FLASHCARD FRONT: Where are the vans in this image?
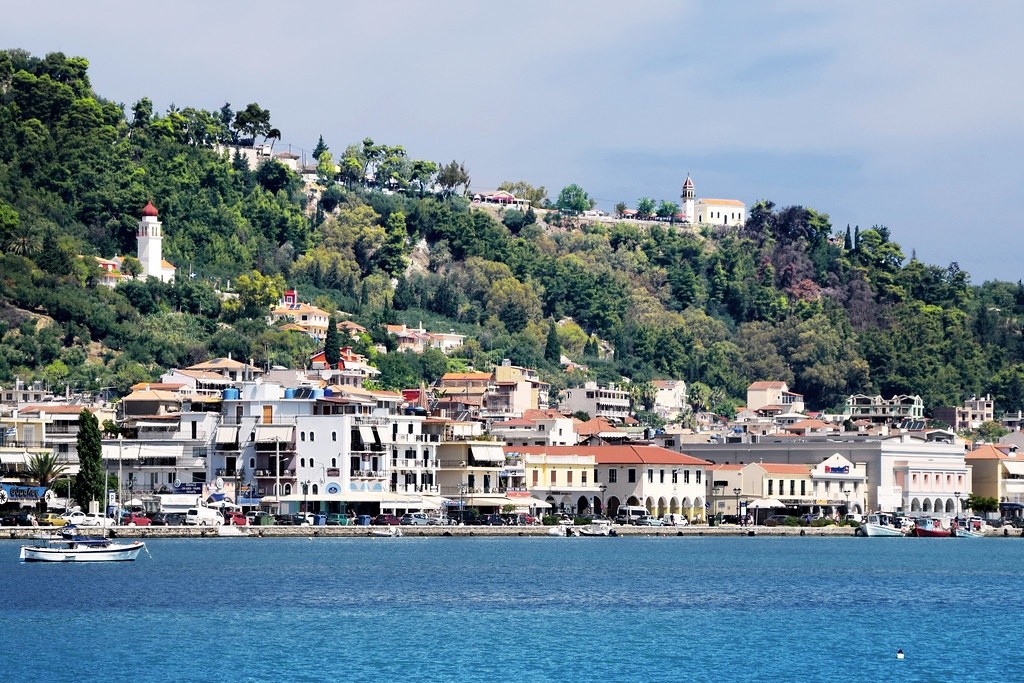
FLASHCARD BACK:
[186,507,225,526]
[664,513,688,527]
[616,505,651,526]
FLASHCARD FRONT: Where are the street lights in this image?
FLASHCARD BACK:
[844,490,850,512]
[733,488,741,525]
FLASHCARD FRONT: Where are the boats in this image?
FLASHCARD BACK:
[859,509,1024,539]
[18,469,146,562]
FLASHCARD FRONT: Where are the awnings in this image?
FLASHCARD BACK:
[999,503,1024,511]
[424,497,552,508]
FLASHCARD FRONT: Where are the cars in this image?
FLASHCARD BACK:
[429,513,457,526]
[374,513,401,526]
[763,514,789,526]
[637,515,665,526]
[37,513,69,526]
[122,512,152,526]
[448,509,616,526]
[800,512,863,526]
[60,511,86,526]
[83,512,117,527]
[245,511,274,525]
[400,513,431,526]
[151,513,184,526]
[275,512,352,526]
[224,512,253,525]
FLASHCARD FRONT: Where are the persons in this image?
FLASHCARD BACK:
[805,514,811,527]
[345,508,356,525]
[25,512,39,526]
[696,512,701,523]
[739,513,755,527]
[835,514,841,526]
[670,512,675,526]
